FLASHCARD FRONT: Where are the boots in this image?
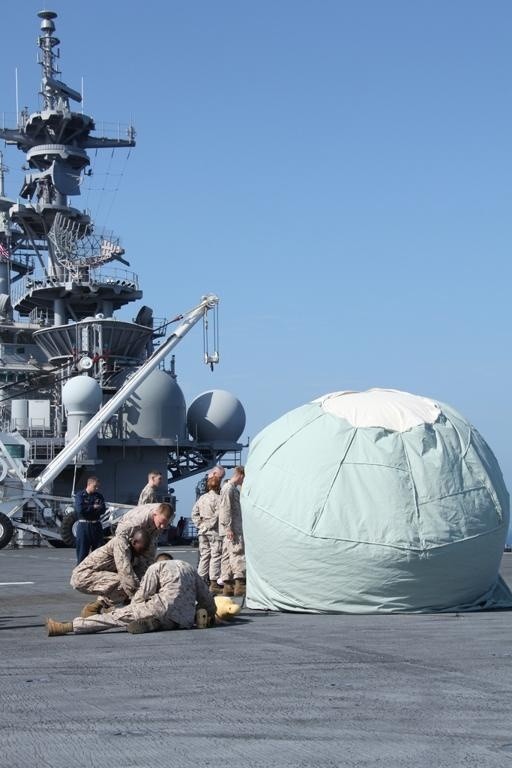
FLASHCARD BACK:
[127,617,161,634]
[46,616,73,636]
[80,601,101,618]
[209,580,247,596]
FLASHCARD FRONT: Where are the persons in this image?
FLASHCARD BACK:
[71,475,106,567]
[219,464,247,599]
[192,466,227,571]
[68,527,152,620]
[43,552,218,638]
[189,476,225,595]
[111,501,175,564]
[137,470,164,505]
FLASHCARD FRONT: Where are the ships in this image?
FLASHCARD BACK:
[0,8,512,768]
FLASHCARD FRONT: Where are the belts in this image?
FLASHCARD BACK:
[79,519,101,524]
